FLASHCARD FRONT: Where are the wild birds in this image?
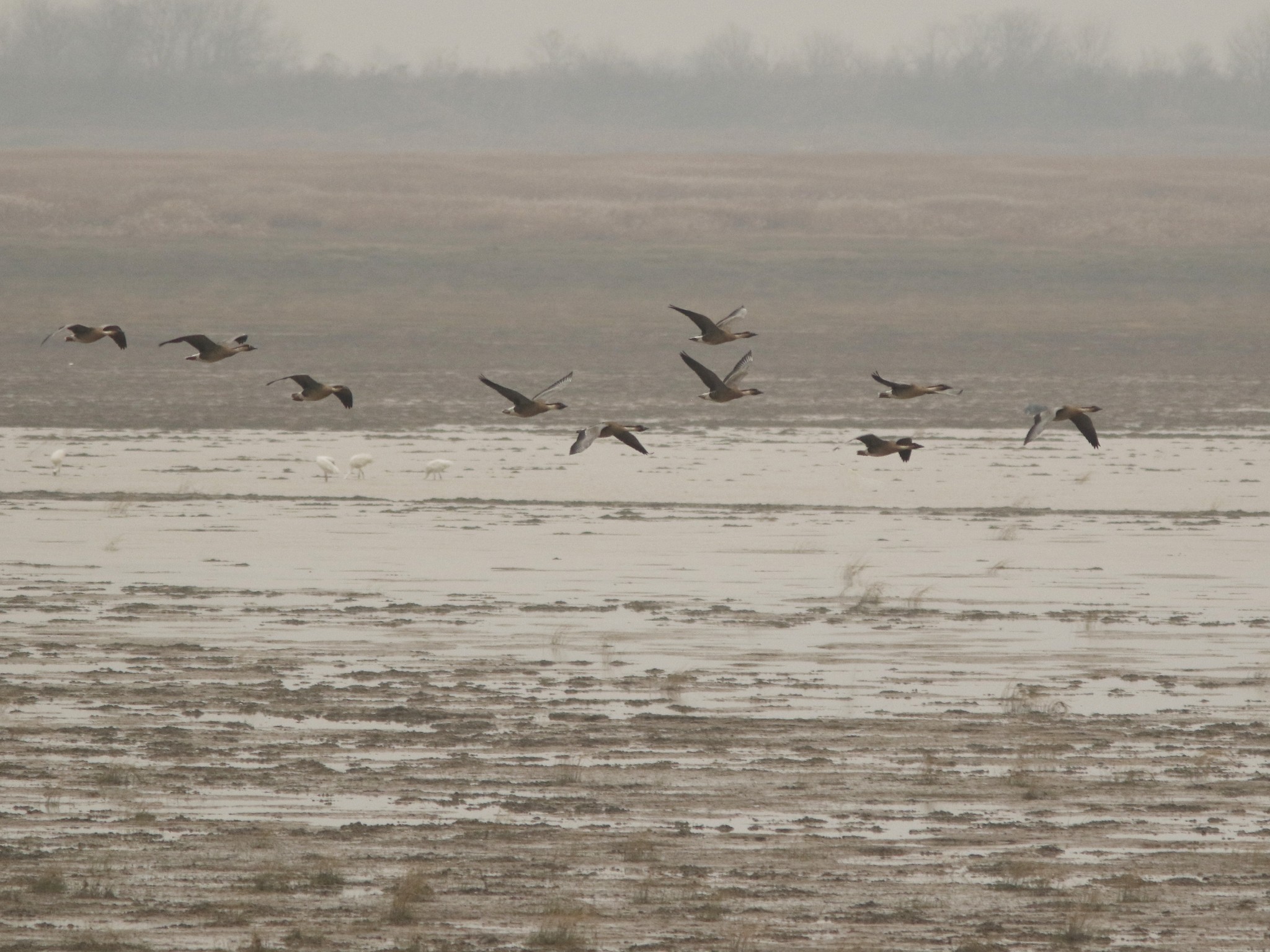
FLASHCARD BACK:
[478,371,573,418]
[835,433,923,461]
[569,421,653,455]
[870,371,965,398]
[680,350,763,402]
[159,334,257,362]
[1021,402,1104,448]
[316,453,375,481]
[51,449,64,476]
[266,374,353,409]
[41,323,128,350]
[668,304,758,345]
[423,459,454,479]
